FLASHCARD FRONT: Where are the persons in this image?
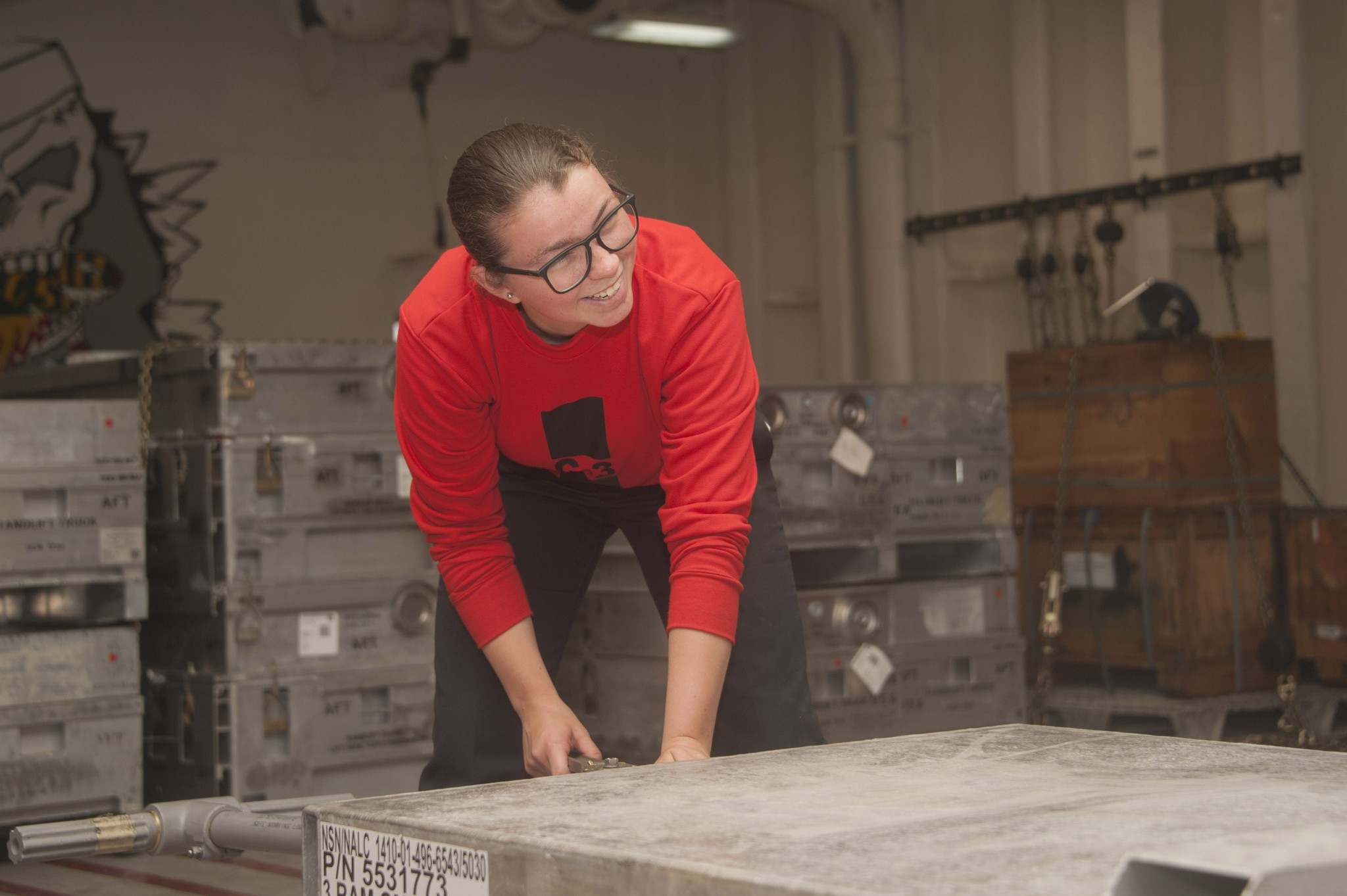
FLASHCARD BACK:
[398,125,815,791]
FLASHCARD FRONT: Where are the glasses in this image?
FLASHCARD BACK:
[500,187,638,294]
